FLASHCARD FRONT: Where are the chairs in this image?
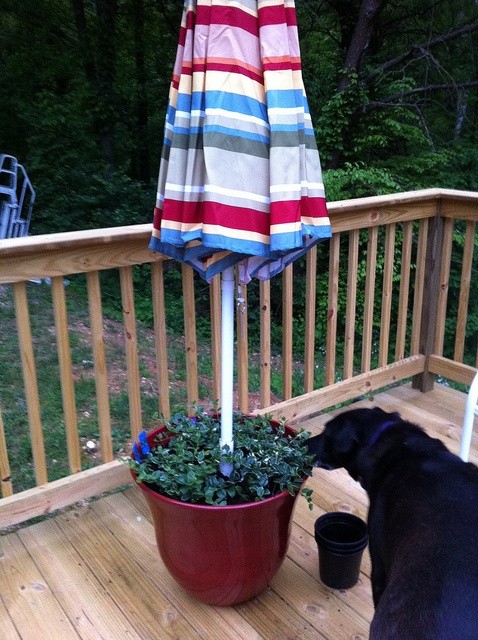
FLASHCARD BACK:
[460,371,478,464]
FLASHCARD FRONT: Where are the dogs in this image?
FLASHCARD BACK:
[300,408,478,640]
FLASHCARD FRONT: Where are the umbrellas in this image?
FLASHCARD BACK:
[147,1,333,480]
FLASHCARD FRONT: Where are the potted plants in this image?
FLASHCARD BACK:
[120,384,324,608]
